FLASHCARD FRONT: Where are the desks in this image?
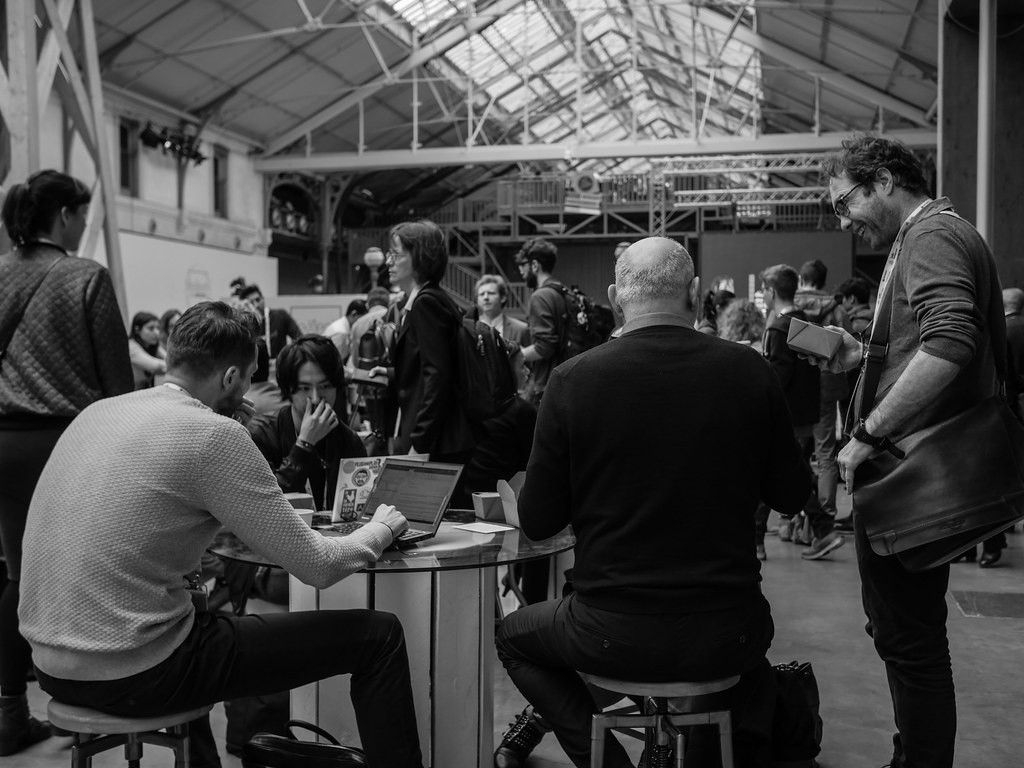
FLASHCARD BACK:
[204,503,576,768]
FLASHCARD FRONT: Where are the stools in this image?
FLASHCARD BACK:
[579,670,742,768]
[46,697,213,768]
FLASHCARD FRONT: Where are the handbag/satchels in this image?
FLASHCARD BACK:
[853,395,1023,569]
[691,662,822,768]
[244,720,368,768]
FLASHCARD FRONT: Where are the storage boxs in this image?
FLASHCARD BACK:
[471,469,526,529]
[780,317,843,362]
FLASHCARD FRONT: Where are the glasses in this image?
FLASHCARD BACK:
[385,251,411,263]
[834,178,867,219]
[518,258,530,269]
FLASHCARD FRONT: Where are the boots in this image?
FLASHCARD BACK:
[1,694,52,757]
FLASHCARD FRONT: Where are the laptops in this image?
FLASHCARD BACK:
[306,452,466,552]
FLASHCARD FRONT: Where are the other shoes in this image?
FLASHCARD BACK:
[225,739,245,758]
[755,545,768,562]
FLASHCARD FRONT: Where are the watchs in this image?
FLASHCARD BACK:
[852,418,905,460]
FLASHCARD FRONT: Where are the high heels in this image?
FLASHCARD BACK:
[953,546,978,563]
[502,573,520,596]
[980,532,1008,568]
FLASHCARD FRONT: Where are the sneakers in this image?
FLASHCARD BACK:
[801,531,846,560]
[494,704,551,768]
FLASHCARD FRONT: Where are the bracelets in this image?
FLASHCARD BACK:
[296,439,314,450]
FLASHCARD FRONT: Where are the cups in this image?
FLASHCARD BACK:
[736,340,751,347]
[295,508,314,529]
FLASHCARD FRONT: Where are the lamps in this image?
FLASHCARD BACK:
[139,121,207,166]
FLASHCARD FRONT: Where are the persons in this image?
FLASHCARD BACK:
[322,286,397,370]
[495,238,818,768]
[951,287,1024,567]
[465,273,528,343]
[248,333,369,511]
[821,135,1024,768]
[0,169,135,756]
[514,238,576,405]
[383,219,484,508]
[15,301,424,768]
[699,259,874,560]
[127,310,182,391]
[240,285,302,383]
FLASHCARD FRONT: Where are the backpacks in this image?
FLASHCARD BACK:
[546,284,613,351]
[418,289,517,421]
